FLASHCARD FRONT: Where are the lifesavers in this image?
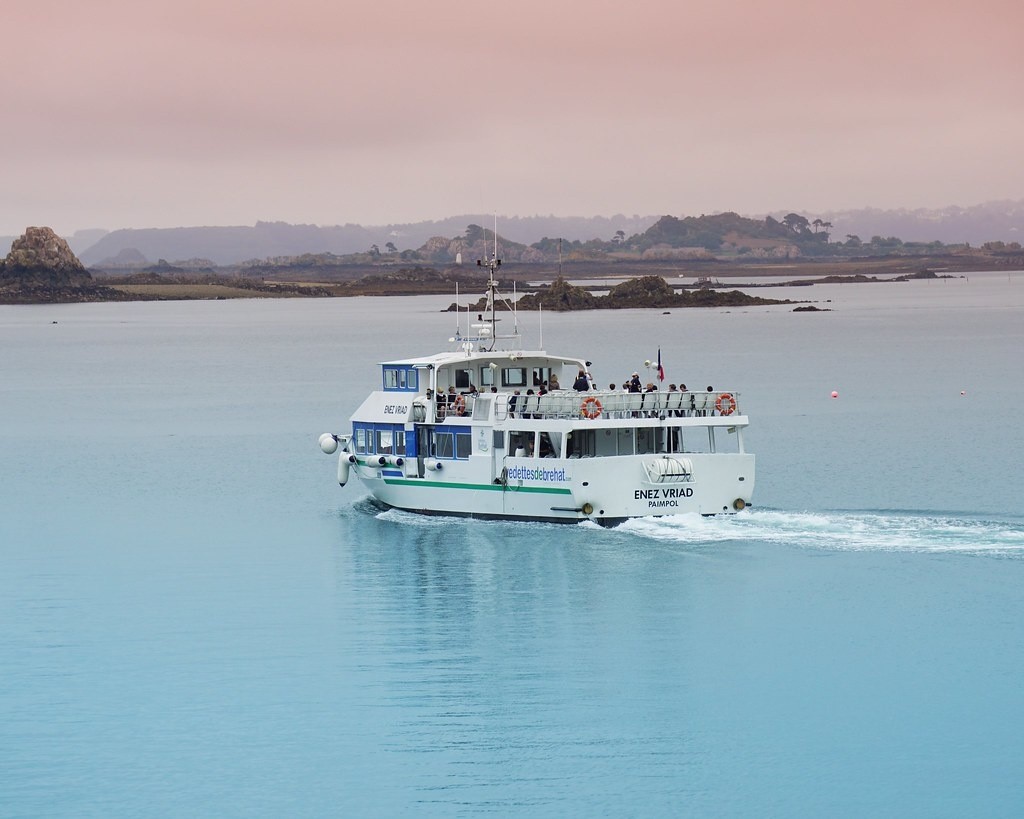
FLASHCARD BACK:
[454,396,466,415]
[581,397,602,418]
[715,393,736,415]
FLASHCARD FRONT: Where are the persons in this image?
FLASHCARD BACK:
[437,369,718,458]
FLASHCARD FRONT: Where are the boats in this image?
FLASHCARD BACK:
[317,210,757,531]
